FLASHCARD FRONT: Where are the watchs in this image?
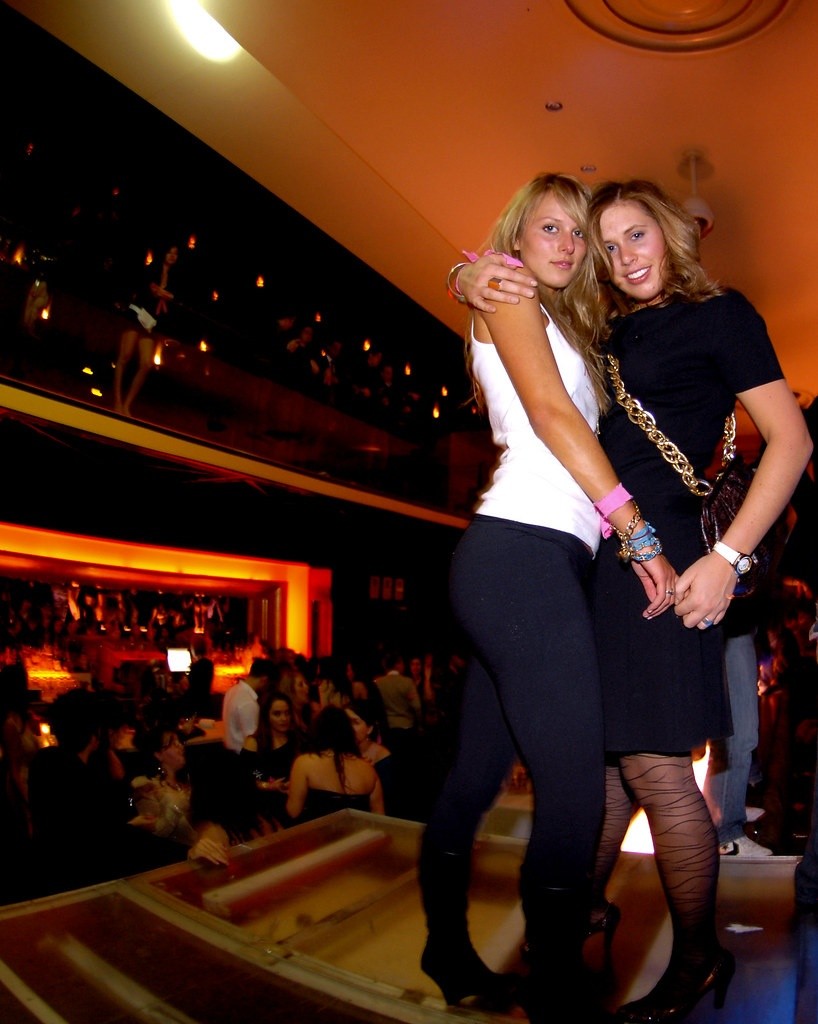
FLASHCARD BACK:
[712,541,752,576]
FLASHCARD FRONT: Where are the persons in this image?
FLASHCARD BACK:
[109,245,418,465]
[455,178,813,1024]
[0,640,421,907]
[418,173,690,1024]
[703,577,817,857]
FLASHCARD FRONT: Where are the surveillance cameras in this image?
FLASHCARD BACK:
[678,197,715,234]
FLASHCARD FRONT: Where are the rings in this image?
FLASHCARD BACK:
[488,278,501,291]
[702,617,712,626]
[666,591,674,595]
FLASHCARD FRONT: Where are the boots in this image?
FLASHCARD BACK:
[418,842,523,1005]
[517,891,624,1024]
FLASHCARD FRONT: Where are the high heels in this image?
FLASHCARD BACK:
[520,903,621,954]
[615,952,735,1024]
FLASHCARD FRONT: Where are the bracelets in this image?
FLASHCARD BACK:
[613,500,662,563]
[594,482,633,539]
[448,263,467,303]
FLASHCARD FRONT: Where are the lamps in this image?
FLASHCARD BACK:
[679,148,715,231]
[564,0,788,54]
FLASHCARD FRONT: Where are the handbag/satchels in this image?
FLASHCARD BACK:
[701,454,790,599]
